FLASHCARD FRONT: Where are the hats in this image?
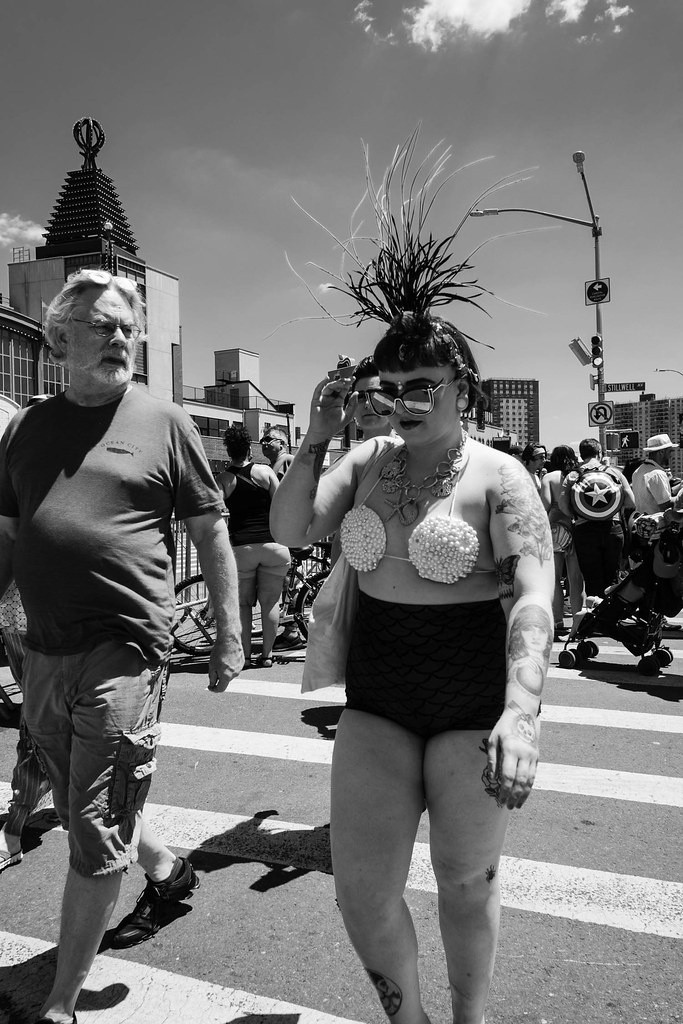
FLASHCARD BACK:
[643,434,679,452]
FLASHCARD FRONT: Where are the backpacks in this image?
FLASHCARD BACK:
[568,463,626,521]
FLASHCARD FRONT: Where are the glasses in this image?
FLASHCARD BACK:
[66,316,141,341]
[88,270,138,291]
[260,437,282,444]
[365,374,469,417]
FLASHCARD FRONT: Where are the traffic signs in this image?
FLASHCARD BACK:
[607,382,646,392]
[585,278,610,305]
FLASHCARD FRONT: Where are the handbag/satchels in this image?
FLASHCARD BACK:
[302,436,396,693]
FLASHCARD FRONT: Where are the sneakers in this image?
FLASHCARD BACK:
[112,856,200,950]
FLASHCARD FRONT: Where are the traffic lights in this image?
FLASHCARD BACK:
[591,334,604,368]
[619,432,640,450]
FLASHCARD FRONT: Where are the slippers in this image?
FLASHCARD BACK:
[0,848,24,871]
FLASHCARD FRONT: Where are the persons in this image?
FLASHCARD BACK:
[270,316,554,1024]
[214,424,292,669]
[511,432,683,642]
[0,268,247,1024]
[260,428,303,650]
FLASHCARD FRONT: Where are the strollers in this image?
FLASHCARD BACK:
[558,520,683,676]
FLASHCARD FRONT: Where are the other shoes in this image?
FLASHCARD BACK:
[553,620,563,631]
[242,657,252,668]
[273,633,303,651]
[257,654,274,667]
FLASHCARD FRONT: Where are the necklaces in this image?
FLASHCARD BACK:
[381,428,469,527]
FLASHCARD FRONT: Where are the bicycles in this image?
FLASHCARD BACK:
[171,541,332,657]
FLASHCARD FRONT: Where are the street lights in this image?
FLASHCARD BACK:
[468,150,607,458]
